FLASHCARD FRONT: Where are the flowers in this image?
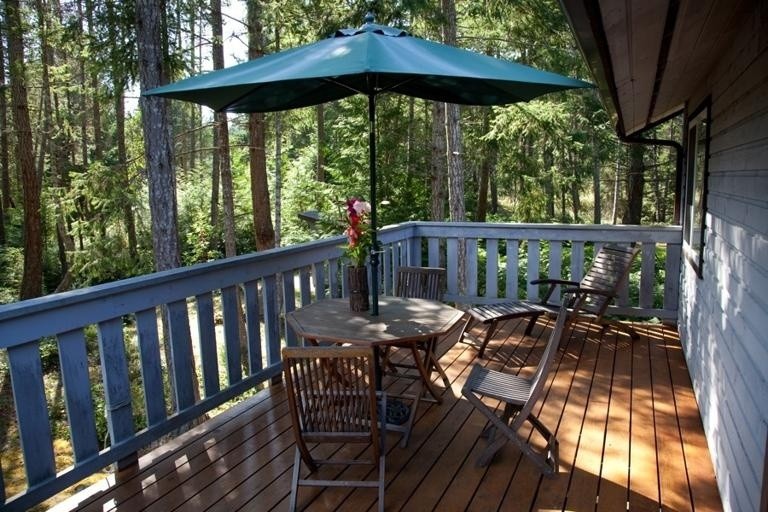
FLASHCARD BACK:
[343,195,373,268]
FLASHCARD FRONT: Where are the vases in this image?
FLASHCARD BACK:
[346,265,370,312]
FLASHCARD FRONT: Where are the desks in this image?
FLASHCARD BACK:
[284,294,465,450]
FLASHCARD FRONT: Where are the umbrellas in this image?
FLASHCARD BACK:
[135,13,597,400]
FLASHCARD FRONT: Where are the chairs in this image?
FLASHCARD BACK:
[461,305,567,481]
[376,266,448,401]
[458,245,642,358]
[282,344,387,512]
